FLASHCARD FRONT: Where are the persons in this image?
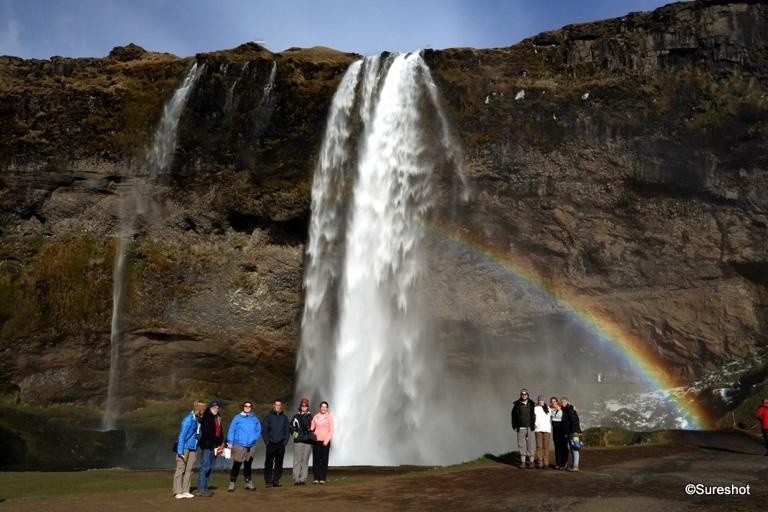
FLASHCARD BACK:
[560,399,581,472]
[754,397,767,457]
[289,399,313,485]
[510,389,534,469]
[225,400,262,492]
[532,396,551,469]
[260,399,290,488]
[197,401,219,498]
[312,402,333,484]
[548,397,576,470]
[172,401,207,499]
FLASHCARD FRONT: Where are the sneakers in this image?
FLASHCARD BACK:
[174,480,326,499]
[518,462,579,472]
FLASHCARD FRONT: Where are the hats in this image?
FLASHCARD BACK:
[210,399,309,407]
[519,388,569,401]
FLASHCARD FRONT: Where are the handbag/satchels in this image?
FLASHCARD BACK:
[172,441,178,452]
